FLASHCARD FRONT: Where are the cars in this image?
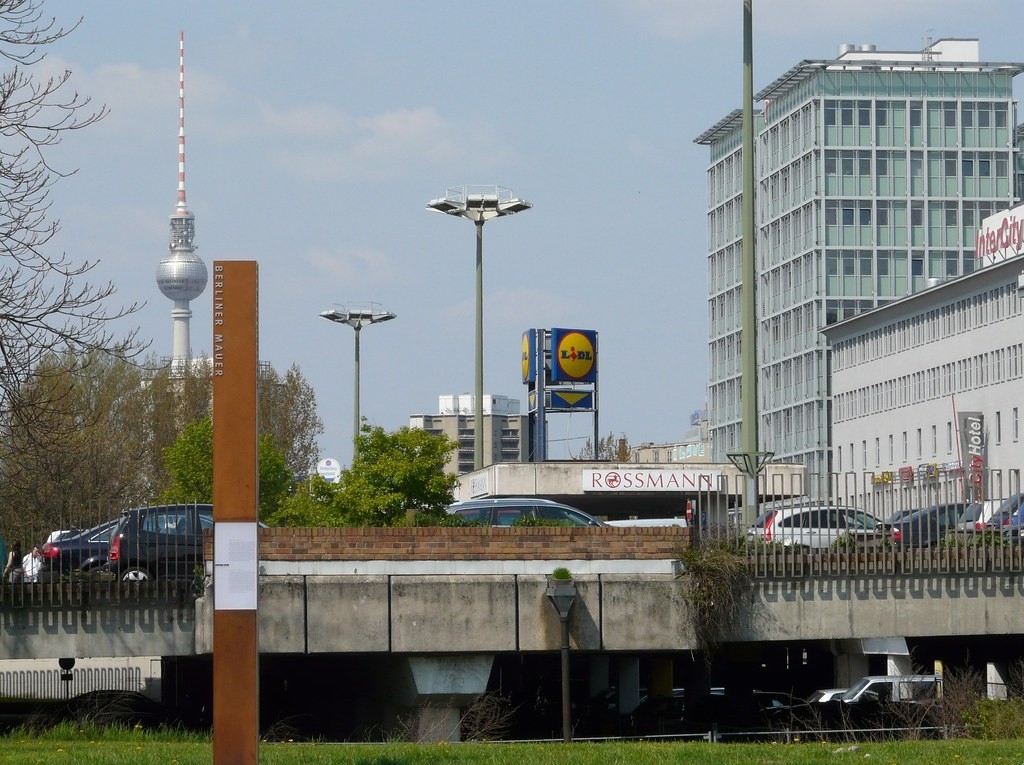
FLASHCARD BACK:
[438,499,621,528]
[747,495,1024,544]
[594,675,945,732]
[40,502,271,586]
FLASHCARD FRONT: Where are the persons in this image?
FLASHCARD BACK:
[23,546,42,582]
[3,538,24,582]
